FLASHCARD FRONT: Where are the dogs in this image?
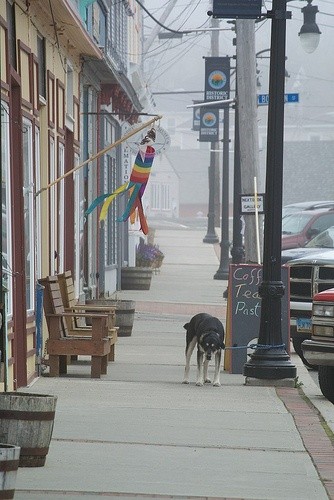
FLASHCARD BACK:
[183,312,225,386]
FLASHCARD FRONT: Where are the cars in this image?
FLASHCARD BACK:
[334,226,334,266]
[284,250,334,372]
[282,201,334,217]
[302,287,334,401]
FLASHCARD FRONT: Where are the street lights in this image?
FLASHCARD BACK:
[224,49,288,299]
[244,1,324,379]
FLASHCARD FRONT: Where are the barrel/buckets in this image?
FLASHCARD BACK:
[121,267,153,290]
[0,392,58,467]
[0,442,21,500]
[86,300,136,337]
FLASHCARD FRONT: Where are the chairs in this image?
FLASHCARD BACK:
[39,269,119,380]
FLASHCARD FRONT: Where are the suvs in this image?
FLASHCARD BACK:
[282,211,334,248]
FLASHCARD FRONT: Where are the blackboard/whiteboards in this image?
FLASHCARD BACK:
[225,263,290,375]
